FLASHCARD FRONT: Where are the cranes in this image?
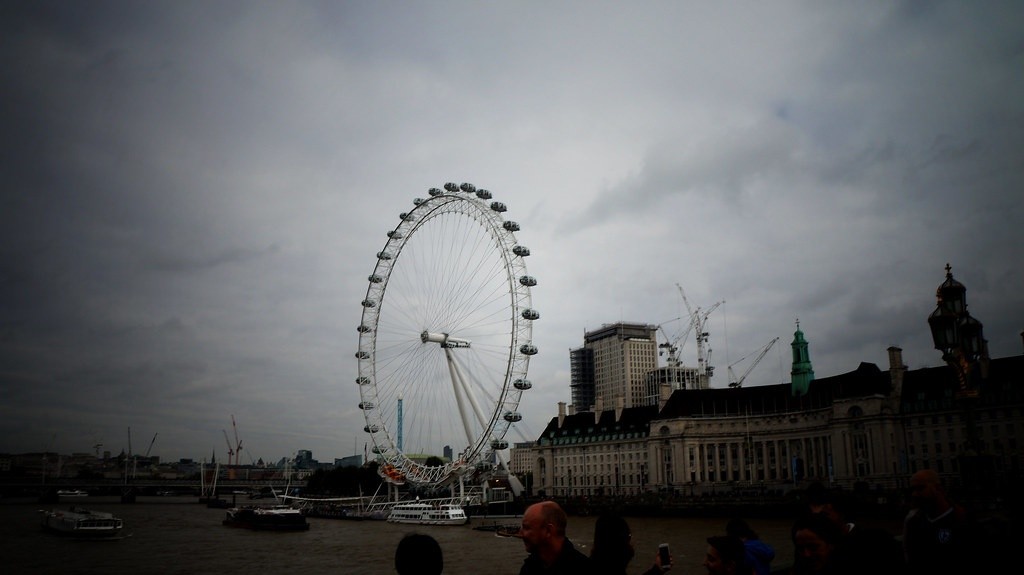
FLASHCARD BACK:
[656,283,779,394]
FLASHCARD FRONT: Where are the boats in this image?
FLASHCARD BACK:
[469,501,529,530]
[387,496,468,525]
[222,504,310,532]
[35,507,134,542]
[269,483,397,521]
[57,489,88,503]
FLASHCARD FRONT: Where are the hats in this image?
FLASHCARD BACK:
[911,469,940,497]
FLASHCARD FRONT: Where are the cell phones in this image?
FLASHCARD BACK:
[659,543,672,569]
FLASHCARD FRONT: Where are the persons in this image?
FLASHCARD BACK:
[703,518,776,575]
[588,515,675,575]
[395,534,443,575]
[790,481,908,575]
[901,470,974,575]
[517,500,602,575]
